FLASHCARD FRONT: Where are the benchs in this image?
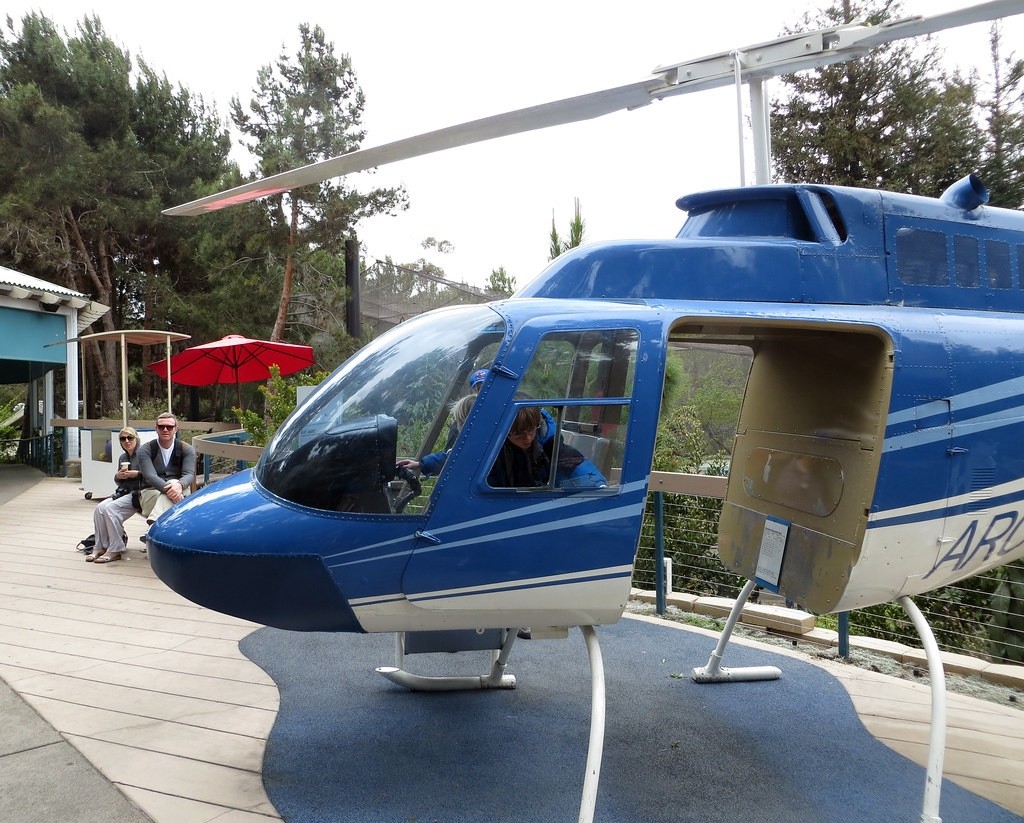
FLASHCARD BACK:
[192,473,232,492]
[559,427,616,481]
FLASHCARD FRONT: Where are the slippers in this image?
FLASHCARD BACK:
[94,555,121,563]
[86,552,102,562]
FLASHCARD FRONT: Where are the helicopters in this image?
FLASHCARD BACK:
[140,0,1022,823]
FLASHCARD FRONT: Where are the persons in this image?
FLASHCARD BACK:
[84,426,144,564]
[390,367,613,489]
[135,412,197,551]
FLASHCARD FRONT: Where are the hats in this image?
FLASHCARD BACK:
[469,369,490,389]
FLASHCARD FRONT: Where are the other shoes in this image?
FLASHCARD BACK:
[140,535,146,543]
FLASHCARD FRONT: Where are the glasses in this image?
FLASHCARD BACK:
[120,436,134,441]
[507,424,542,439]
[157,425,175,431]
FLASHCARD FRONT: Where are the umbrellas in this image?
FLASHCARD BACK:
[147,331,316,428]
